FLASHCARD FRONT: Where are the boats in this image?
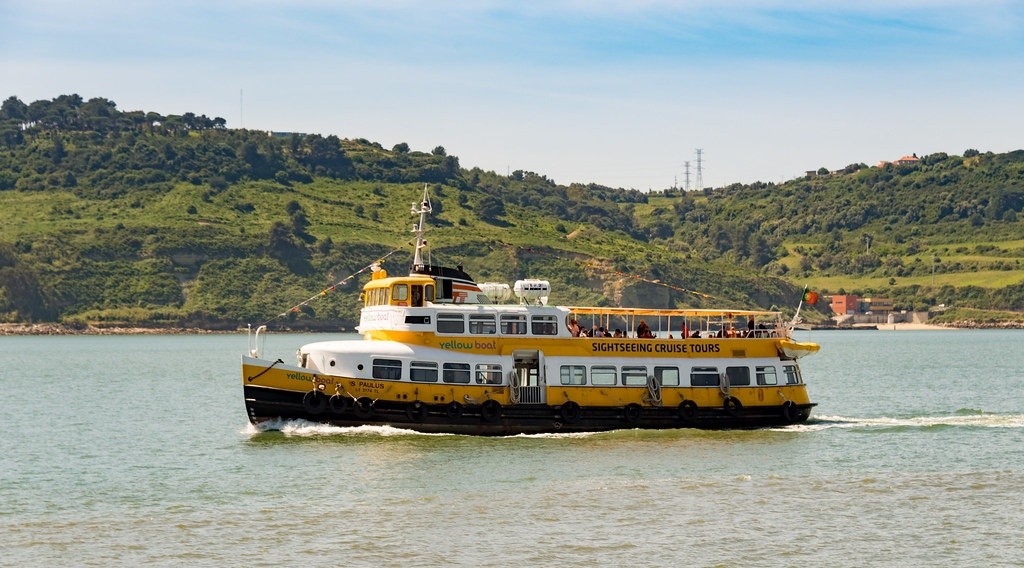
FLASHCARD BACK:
[242,184,820,437]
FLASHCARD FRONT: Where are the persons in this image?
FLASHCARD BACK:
[717,325,735,338]
[742,317,766,338]
[691,331,701,338]
[636,320,657,339]
[681,322,689,339]
[570,319,629,338]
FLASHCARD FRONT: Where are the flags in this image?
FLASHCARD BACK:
[802,289,819,304]
